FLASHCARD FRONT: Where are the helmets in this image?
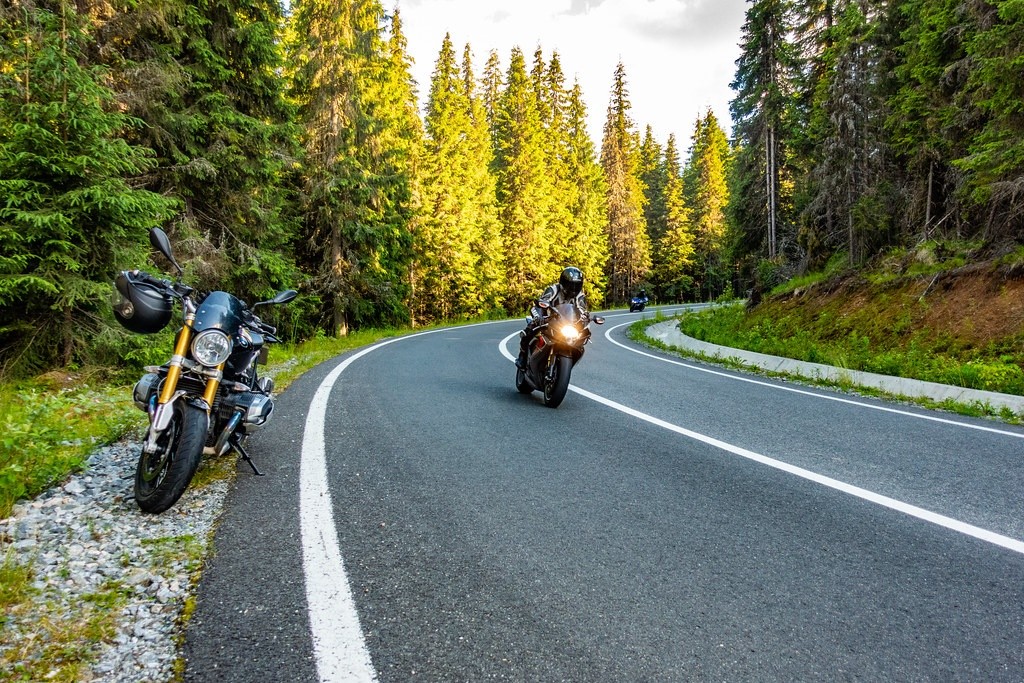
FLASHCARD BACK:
[113,271,173,334]
[641,289,645,293]
[560,267,584,298]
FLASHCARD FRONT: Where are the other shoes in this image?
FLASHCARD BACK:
[516,356,528,371]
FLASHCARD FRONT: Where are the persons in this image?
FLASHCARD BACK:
[637,289,646,309]
[517,267,591,371]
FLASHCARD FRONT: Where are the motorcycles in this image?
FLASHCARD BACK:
[514,297,605,408]
[134,227,299,514]
[629,296,649,313]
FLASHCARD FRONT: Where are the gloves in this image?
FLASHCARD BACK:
[534,315,544,326]
